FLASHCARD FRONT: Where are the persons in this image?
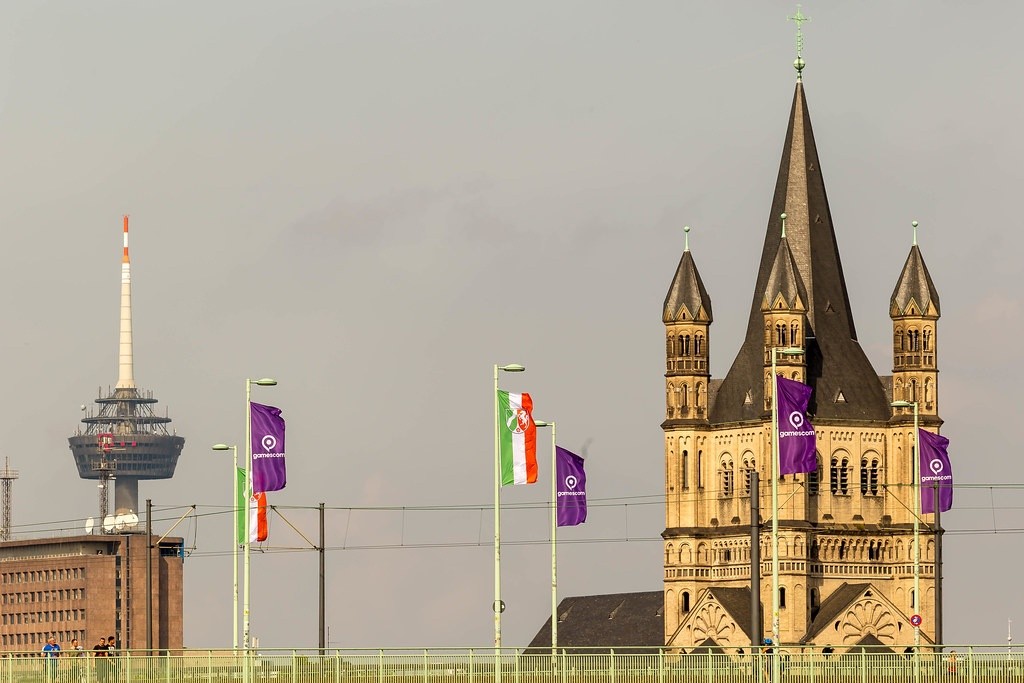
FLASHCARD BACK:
[69,636,119,683]
[947,651,958,676]
[41,637,61,683]
[761,639,781,683]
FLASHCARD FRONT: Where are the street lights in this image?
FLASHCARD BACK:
[535,419,557,668]
[493,363,526,682]
[242,378,277,683]
[212,444,239,657]
[771,345,805,683]
[890,401,920,682]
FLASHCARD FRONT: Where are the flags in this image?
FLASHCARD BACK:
[236,467,267,545]
[249,401,287,496]
[919,428,953,515]
[497,389,538,488]
[776,374,817,476]
[556,445,587,527]
